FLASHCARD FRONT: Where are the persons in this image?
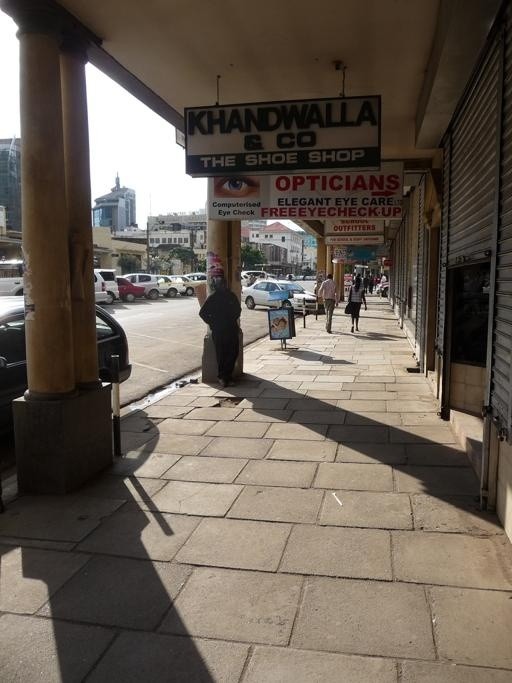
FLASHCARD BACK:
[318,274,341,332]
[198,276,243,388]
[271,317,279,328]
[344,270,386,294]
[271,316,287,332]
[213,174,260,198]
[348,275,367,332]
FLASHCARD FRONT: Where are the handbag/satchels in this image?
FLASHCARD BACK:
[345,302,351,313]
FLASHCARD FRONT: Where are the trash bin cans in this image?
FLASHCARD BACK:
[267,290,296,350]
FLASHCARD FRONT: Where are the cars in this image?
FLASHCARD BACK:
[0,259,206,304]
[241,279,318,313]
[241,271,316,286]
[0,301,132,419]
